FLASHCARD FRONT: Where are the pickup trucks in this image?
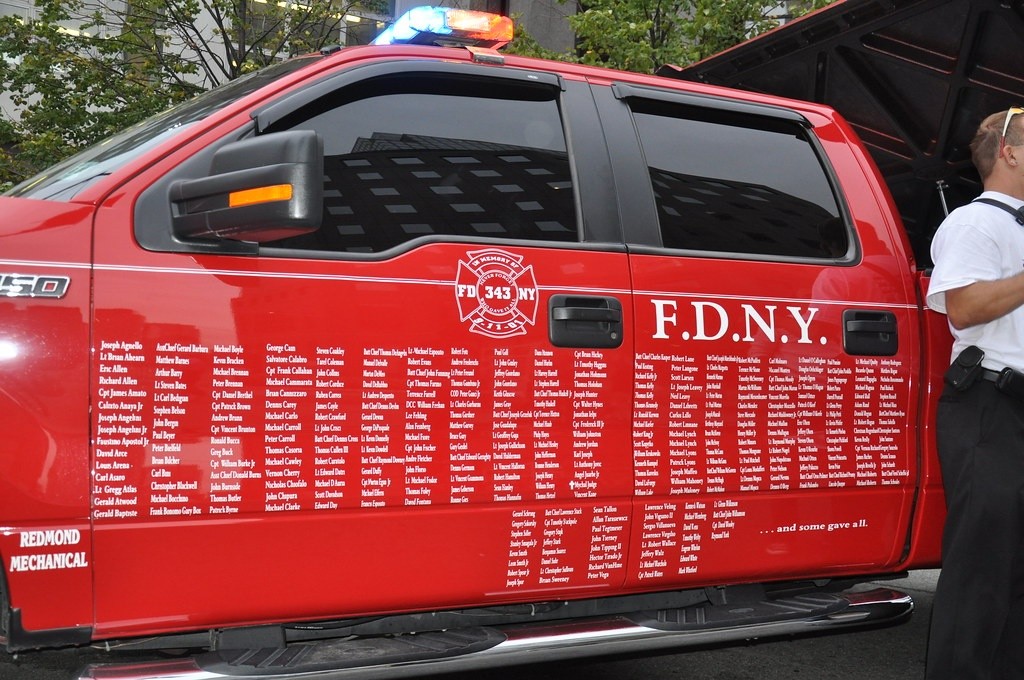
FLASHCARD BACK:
[0,4,953,680]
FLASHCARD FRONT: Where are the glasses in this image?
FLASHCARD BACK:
[998,106,1024,158]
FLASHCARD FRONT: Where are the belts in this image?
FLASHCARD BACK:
[976,369,1000,381]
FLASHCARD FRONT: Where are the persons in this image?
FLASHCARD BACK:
[920,103,1023,680]
